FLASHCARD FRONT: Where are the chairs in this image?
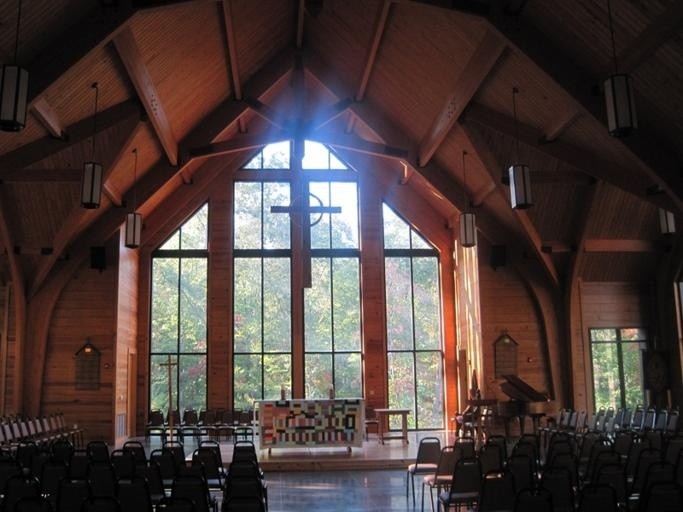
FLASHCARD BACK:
[366,404,381,442]
[0,409,269,512]
[404,401,683,512]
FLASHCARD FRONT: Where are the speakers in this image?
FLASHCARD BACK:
[492,244,505,266]
[90,247,105,270]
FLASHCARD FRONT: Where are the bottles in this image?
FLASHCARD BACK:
[329,384,334,399]
[281,385,286,400]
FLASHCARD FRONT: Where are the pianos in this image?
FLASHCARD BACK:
[498,375,551,445]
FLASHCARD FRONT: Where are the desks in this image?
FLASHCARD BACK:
[253,398,366,447]
[374,407,412,446]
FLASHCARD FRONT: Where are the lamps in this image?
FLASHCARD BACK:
[458,148,480,248]
[602,0,640,137]
[505,86,536,211]
[78,82,105,210]
[655,204,677,238]
[0,0,30,134]
[123,147,143,249]
